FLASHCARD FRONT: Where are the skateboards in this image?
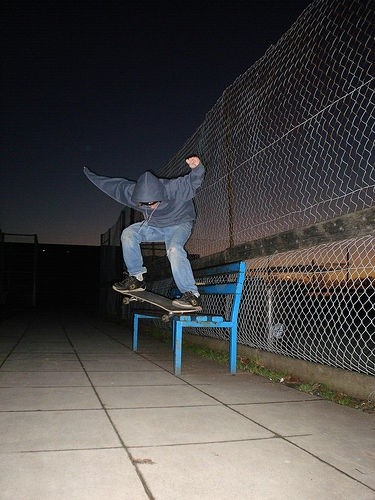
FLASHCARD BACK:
[113,283,202,322]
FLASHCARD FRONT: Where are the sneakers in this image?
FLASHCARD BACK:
[112,272,147,294]
[172,291,203,311]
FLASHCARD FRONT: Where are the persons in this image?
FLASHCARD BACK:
[82,156,207,310]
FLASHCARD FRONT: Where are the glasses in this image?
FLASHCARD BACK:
[138,202,160,207]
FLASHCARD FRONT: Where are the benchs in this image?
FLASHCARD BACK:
[132,261,247,376]
